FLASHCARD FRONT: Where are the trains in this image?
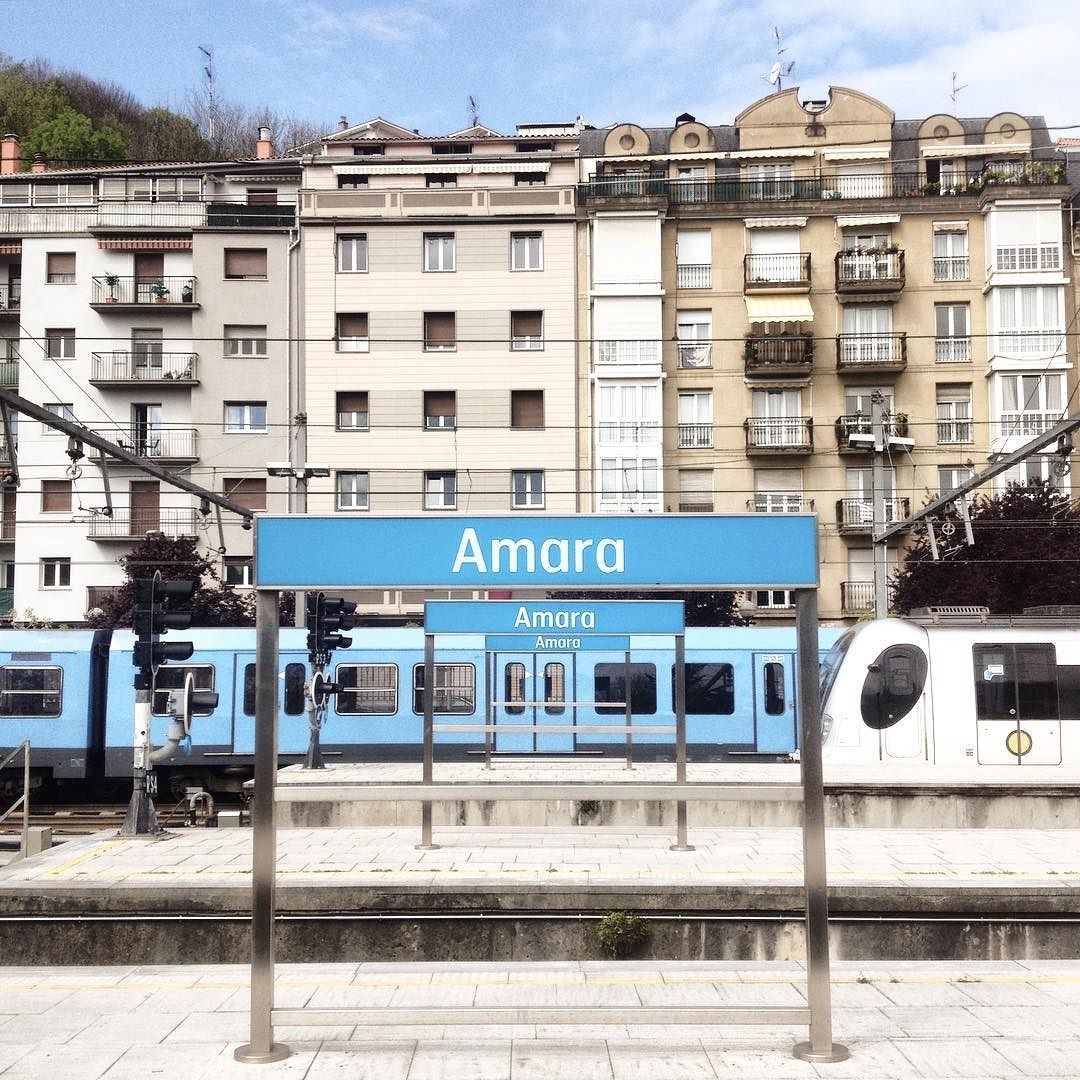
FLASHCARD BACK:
[0,626,844,806]
[798,617,1080,793]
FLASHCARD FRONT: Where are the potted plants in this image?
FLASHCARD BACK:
[105,273,119,304]
[182,280,193,302]
[150,281,170,303]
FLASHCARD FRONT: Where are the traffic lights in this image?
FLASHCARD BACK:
[342,602,359,649]
[150,570,195,677]
[311,671,345,708]
[315,592,346,650]
[183,673,219,735]
